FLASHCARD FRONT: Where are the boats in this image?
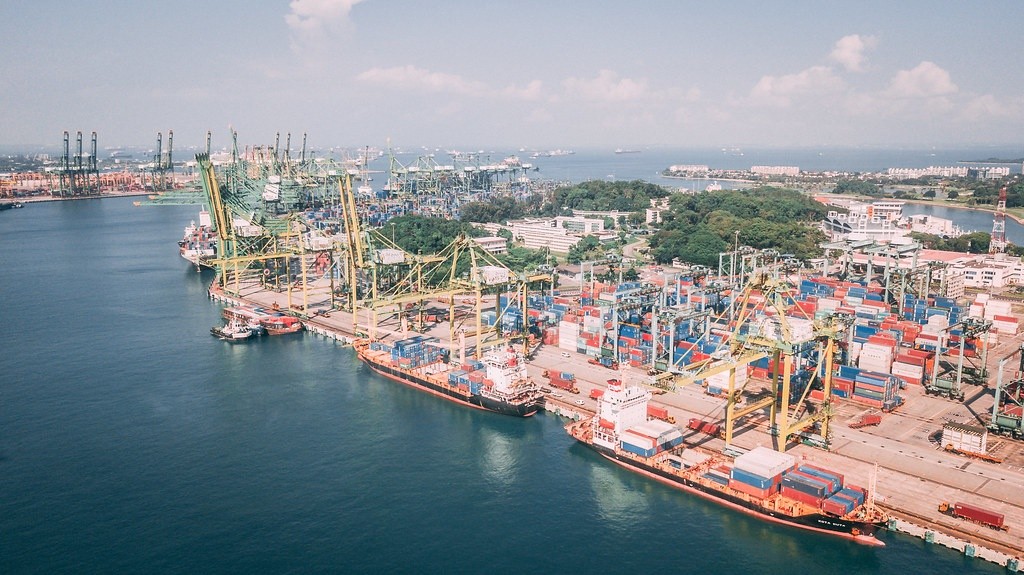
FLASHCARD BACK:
[563,374,890,549]
[351,335,547,417]
[209,307,301,344]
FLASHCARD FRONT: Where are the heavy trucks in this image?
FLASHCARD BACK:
[939,500,1010,533]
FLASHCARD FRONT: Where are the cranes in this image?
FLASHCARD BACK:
[602,242,1024,453]
[54,128,520,306]
